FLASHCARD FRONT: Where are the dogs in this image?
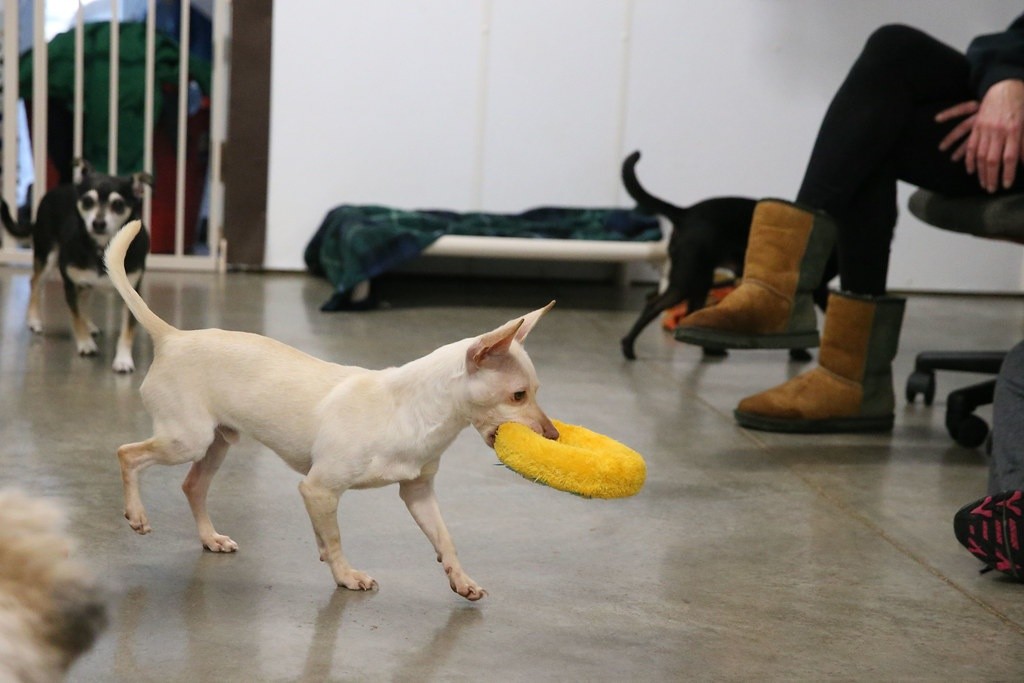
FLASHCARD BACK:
[622,148,850,365]
[103,217,560,604]
[0,156,156,373]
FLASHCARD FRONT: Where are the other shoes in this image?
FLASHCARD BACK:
[953,490,1024,583]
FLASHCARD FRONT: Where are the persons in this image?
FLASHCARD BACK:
[954,341,1024,580]
[675,11,1024,432]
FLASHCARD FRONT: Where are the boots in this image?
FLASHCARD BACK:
[674,197,838,349]
[735,289,908,435]
